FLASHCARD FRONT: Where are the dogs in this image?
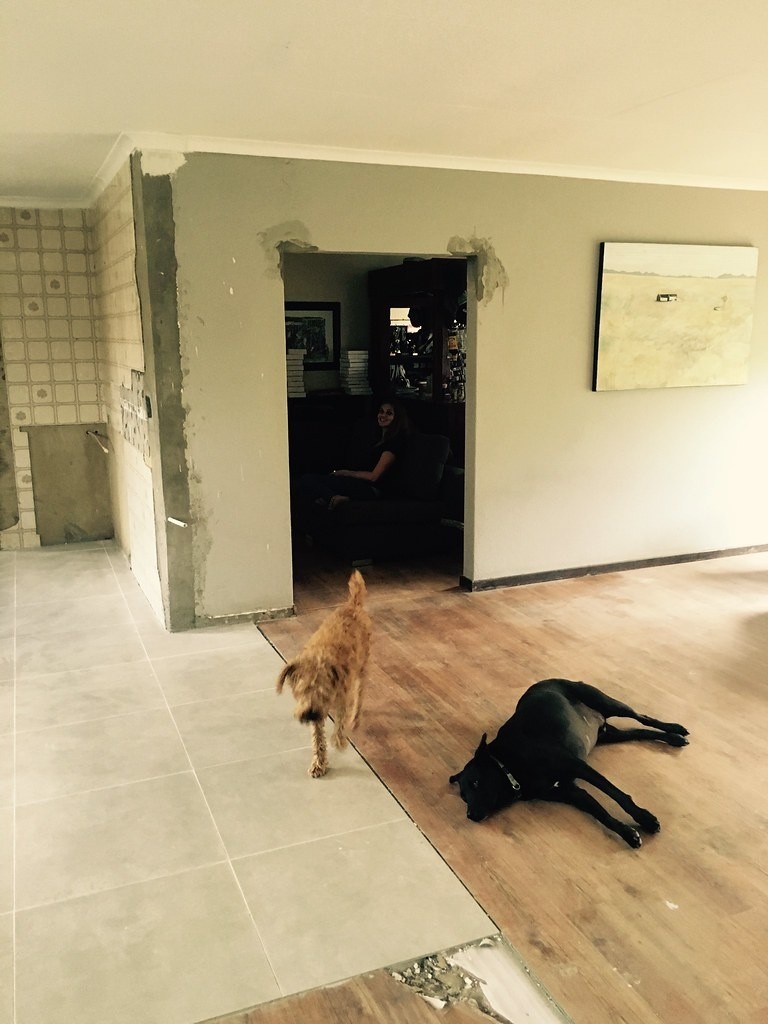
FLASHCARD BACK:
[274,568,373,781]
[448,677,691,851]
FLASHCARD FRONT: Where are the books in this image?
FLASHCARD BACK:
[286,348,309,397]
[339,349,373,396]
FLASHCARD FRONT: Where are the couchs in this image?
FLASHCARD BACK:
[294,433,451,560]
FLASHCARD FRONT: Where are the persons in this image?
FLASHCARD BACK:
[311,398,405,572]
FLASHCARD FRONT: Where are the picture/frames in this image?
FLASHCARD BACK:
[284,300,342,371]
